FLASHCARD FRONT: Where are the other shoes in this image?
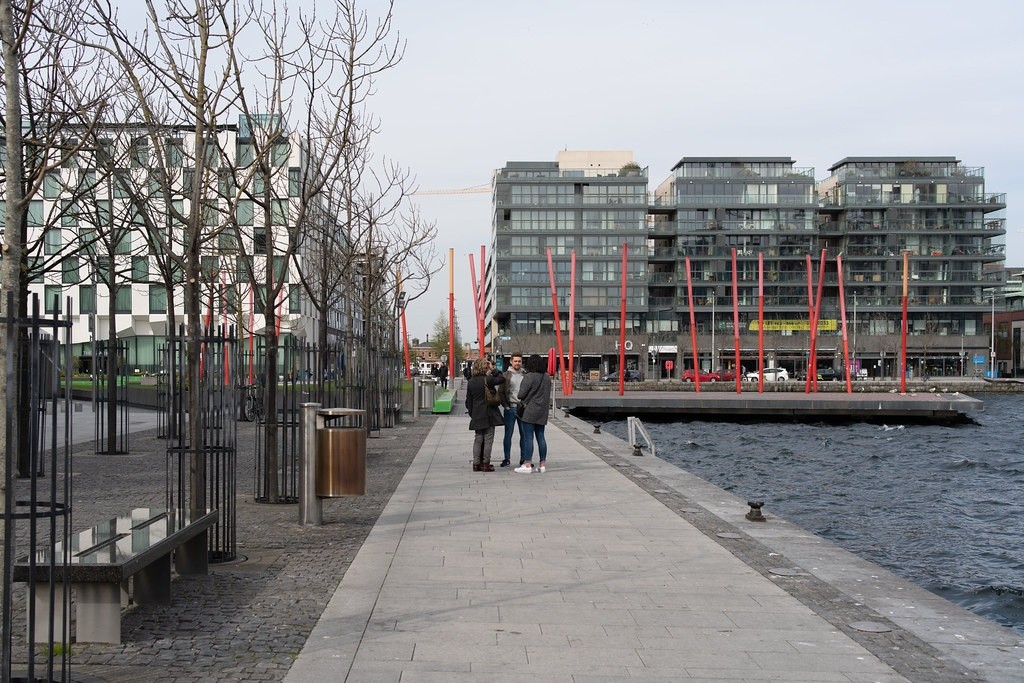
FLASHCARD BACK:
[482,464,495,472]
[473,465,481,471]
[520,460,534,467]
[500,460,510,467]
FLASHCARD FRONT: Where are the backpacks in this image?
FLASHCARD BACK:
[463,368,470,377]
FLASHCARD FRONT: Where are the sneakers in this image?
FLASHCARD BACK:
[514,464,532,474]
[536,465,545,473]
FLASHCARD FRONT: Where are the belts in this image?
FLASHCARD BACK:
[510,404,517,408]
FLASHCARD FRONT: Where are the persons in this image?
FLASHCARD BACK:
[466,361,472,380]
[741,365,748,382]
[431,364,439,385]
[513,354,551,473]
[499,352,534,468]
[487,355,496,369]
[625,368,630,382]
[439,362,448,389]
[465,358,505,471]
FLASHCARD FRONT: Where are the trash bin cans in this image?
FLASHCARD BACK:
[856,368,868,381]
[316,410,368,497]
[418,378,434,410]
[558,368,569,379]
[588,368,600,380]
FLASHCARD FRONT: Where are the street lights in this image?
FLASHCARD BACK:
[983,288,994,378]
[396,290,406,351]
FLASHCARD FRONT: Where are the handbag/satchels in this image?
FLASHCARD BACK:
[517,402,525,417]
[484,377,501,407]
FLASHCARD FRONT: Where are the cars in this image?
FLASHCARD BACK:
[601,370,645,383]
[682,370,720,383]
[711,369,742,382]
[850,371,868,380]
[796,369,841,382]
[743,369,788,382]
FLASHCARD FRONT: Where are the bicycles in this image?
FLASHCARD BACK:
[238,383,265,422]
